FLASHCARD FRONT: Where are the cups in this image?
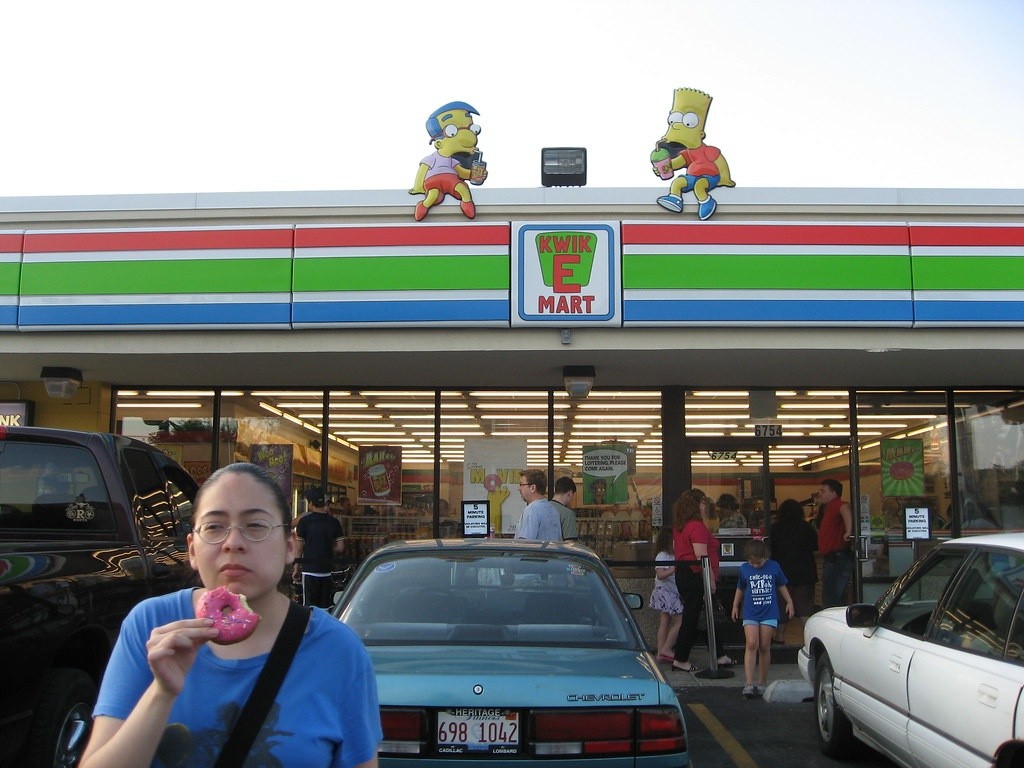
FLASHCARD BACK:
[470,160,486,185]
[369,464,391,496]
[651,157,674,180]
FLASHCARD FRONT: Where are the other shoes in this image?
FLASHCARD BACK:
[741,684,754,695]
[758,685,767,696]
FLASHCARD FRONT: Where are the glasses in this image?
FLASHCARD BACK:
[520,483,532,487]
[192,519,288,544]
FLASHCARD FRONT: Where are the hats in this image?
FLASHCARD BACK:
[302,488,325,507]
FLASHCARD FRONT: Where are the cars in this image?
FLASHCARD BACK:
[798,534,1024,767]
[329,537,694,768]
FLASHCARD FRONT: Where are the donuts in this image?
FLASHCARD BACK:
[196,585,260,645]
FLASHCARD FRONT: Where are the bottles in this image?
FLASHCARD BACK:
[337,534,389,562]
[416,519,433,539]
[389,526,416,540]
[329,503,344,514]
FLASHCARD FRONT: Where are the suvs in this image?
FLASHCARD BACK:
[0,426,201,768]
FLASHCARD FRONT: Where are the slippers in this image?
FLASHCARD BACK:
[771,635,785,644]
[671,662,699,672]
[656,656,673,662]
[658,651,674,661]
[718,659,738,668]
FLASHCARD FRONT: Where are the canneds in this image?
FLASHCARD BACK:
[369,464,391,496]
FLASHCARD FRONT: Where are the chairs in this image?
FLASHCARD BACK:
[970,580,1024,659]
[382,586,468,623]
[32,482,114,529]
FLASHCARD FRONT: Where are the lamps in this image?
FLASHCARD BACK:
[881,403,971,408]
[587,391,663,397]
[39,367,84,399]
[575,402,663,409]
[372,402,470,408]
[145,391,245,398]
[542,147,588,187]
[116,402,203,408]
[474,402,572,410]
[805,390,849,396]
[779,403,850,409]
[688,391,798,397]
[685,403,750,409]
[117,390,141,396]
[277,402,370,408]
[248,391,351,397]
[468,391,570,396]
[563,366,596,399]
[358,391,463,396]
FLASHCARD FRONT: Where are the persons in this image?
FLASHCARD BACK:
[772,498,818,646]
[819,479,854,609]
[716,493,747,561]
[649,529,683,663]
[704,496,720,528]
[291,490,345,610]
[551,476,578,588]
[80,462,384,768]
[732,538,794,697]
[502,469,561,582]
[672,489,740,671]
